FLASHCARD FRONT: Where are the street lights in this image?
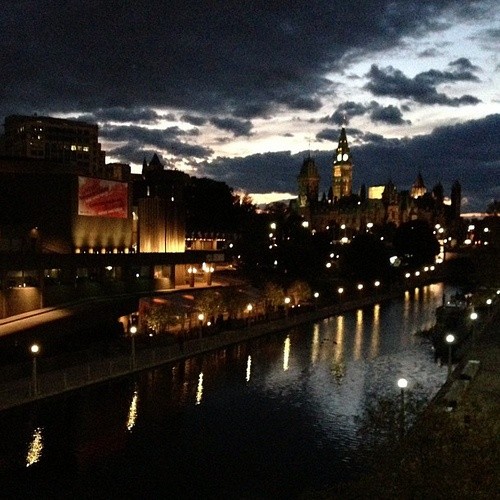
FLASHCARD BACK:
[246,304,253,327]
[446,334,455,385]
[198,314,204,339]
[357,284,363,297]
[284,298,290,318]
[397,378,408,457]
[30,344,39,396]
[313,292,320,312]
[486,299,493,318]
[129,327,138,359]
[337,288,343,305]
[374,281,380,295]
[470,313,478,359]
